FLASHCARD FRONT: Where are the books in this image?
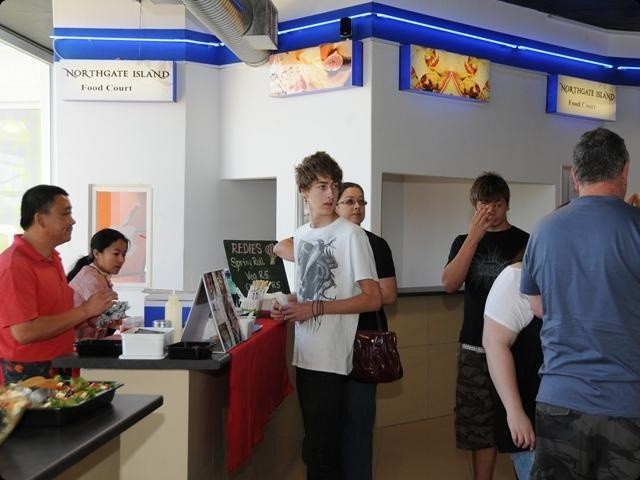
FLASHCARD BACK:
[179,268,247,354]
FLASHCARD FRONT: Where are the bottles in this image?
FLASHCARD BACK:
[165,290,183,345]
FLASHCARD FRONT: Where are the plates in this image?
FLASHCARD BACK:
[25,380,123,426]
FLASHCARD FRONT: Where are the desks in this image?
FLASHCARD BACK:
[53,310,302,480]
[0,393,163,480]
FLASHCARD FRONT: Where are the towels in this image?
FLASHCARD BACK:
[96,301,131,331]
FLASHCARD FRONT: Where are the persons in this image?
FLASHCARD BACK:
[0,185,118,381]
[442,172,531,480]
[270,151,383,480]
[66,228,131,340]
[519,127,639,479]
[482,201,574,480]
[273,182,398,480]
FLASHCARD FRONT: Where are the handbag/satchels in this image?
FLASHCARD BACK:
[354,310,404,384]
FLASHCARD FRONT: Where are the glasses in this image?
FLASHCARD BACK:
[337,199,367,206]
